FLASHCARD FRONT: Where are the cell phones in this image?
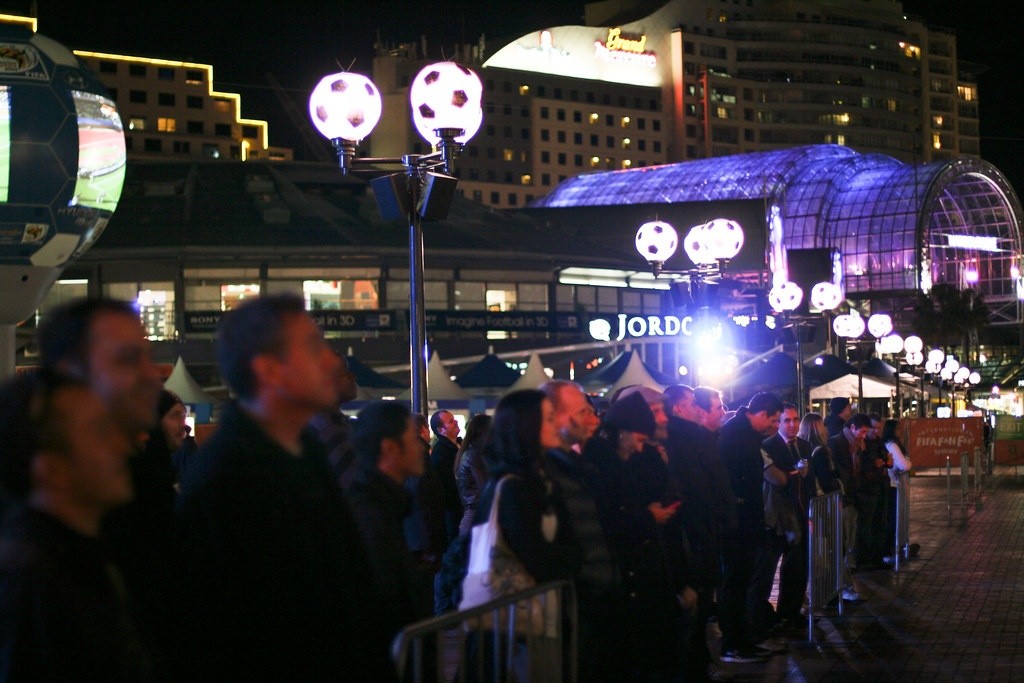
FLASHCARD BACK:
[665,502,681,511]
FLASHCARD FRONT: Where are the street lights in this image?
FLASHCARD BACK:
[769,280,842,420]
[834,313,893,413]
[876,334,982,419]
[634,217,745,386]
[306,63,485,418]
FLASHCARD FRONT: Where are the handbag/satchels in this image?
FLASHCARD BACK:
[460,474,558,638]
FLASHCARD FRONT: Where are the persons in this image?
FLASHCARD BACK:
[32,298,184,536]
[0,374,201,683]
[200,290,394,683]
[153,386,924,683]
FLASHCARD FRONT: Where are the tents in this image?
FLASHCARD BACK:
[342,344,928,404]
[164,356,214,407]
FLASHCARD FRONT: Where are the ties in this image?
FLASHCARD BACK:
[790,440,800,460]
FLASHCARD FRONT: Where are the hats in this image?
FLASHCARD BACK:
[605,390,655,436]
[159,387,187,422]
[612,385,667,404]
[829,398,849,415]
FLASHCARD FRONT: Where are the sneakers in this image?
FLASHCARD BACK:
[720,649,766,662]
[704,664,736,680]
[751,647,771,656]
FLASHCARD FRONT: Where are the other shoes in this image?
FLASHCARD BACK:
[865,562,894,571]
[755,639,785,652]
